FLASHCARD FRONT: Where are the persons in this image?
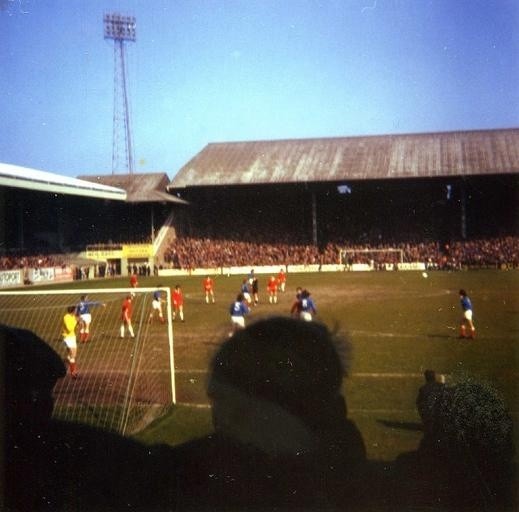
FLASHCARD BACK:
[0,184,519,511]
